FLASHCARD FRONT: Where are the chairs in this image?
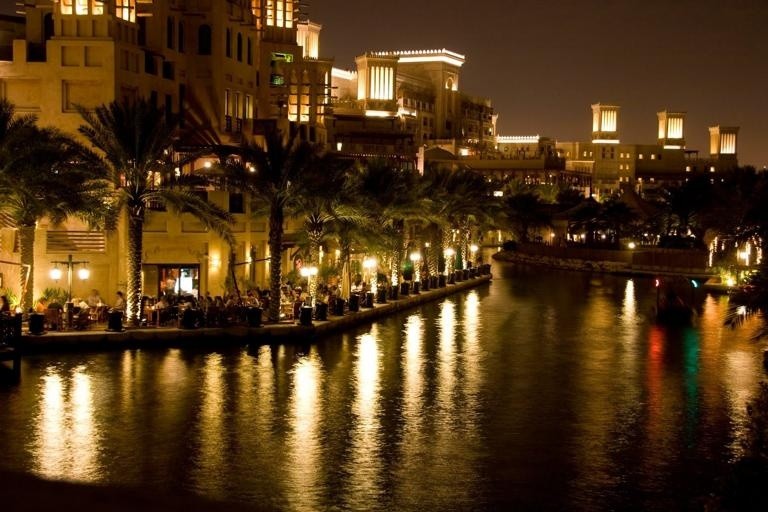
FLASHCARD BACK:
[0,294,299,328]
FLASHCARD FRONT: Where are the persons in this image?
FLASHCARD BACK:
[4,287,127,328]
[141,282,367,329]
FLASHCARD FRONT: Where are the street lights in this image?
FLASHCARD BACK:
[450,228,460,241]
[300,265,319,307]
[49,254,92,333]
[361,259,377,294]
[550,231,555,246]
[444,248,456,280]
[470,244,480,258]
[409,252,421,282]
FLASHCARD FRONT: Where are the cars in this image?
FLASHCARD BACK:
[565,228,696,243]
